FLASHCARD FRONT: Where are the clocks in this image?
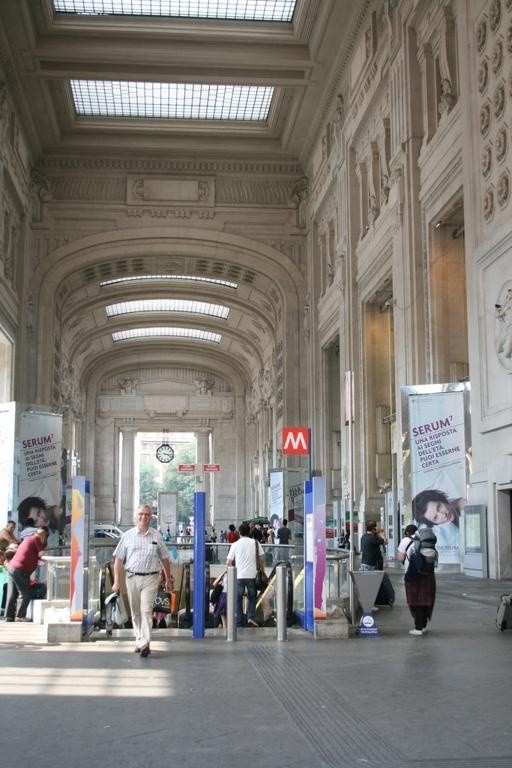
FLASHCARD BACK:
[155,428,174,464]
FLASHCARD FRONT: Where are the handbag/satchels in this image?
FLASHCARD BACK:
[211,584,224,604]
[104,592,128,625]
[154,591,175,612]
[32,583,46,599]
[255,569,268,591]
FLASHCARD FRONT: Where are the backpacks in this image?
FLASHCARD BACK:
[407,528,438,576]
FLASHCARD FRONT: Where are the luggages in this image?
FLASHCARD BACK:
[375,573,395,607]
[495,593,512,632]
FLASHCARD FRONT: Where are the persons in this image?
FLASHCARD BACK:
[412,490,464,529]
[17,497,59,529]
[0,518,47,622]
[154,519,389,629]
[112,505,173,656]
[397,525,435,635]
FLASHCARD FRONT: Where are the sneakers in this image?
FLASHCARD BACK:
[409,629,423,635]
[248,617,259,627]
[15,617,30,622]
[140,648,153,657]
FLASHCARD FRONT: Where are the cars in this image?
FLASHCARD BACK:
[95,532,120,538]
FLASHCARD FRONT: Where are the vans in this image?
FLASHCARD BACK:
[94,525,124,536]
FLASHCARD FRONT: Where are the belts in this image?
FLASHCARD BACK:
[134,571,158,576]
